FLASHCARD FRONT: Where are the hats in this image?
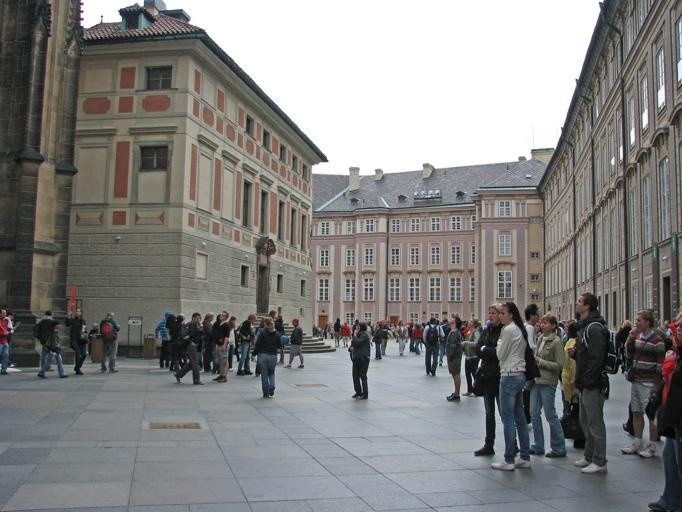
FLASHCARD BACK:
[165,311,172,318]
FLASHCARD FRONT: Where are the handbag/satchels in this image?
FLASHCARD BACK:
[627,367,636,382]
[236,327,250,340]
[525,345,541,381]
[155,338,163,348]
[472,367,484,396]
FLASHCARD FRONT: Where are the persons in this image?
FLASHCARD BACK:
[474,293,609,473]
[6,311,21,368]
[615,310,682,512]
[65,308,87,375]
[38,310,65,378]
[0,309,14,374]
[348,322,370,399]
[155,307,285,398]
[314,324,322,337]
[324,318,395,360]
[88,323,99,356]
[396,315,481,402]
[100,312,121,372]
[285,319,304,367]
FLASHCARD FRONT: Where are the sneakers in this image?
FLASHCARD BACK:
[159,360,304,398]
[352,393,363,398]
[1,361,118,377]
[446,392,680,511]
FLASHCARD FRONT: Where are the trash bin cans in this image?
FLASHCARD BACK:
[143,333,157,360]
[90,334,104,364]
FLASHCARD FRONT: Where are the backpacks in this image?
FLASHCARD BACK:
[428,324,438,346]
[583,322,618,374]
[101,319,114,342]
[441,325,450,341]
